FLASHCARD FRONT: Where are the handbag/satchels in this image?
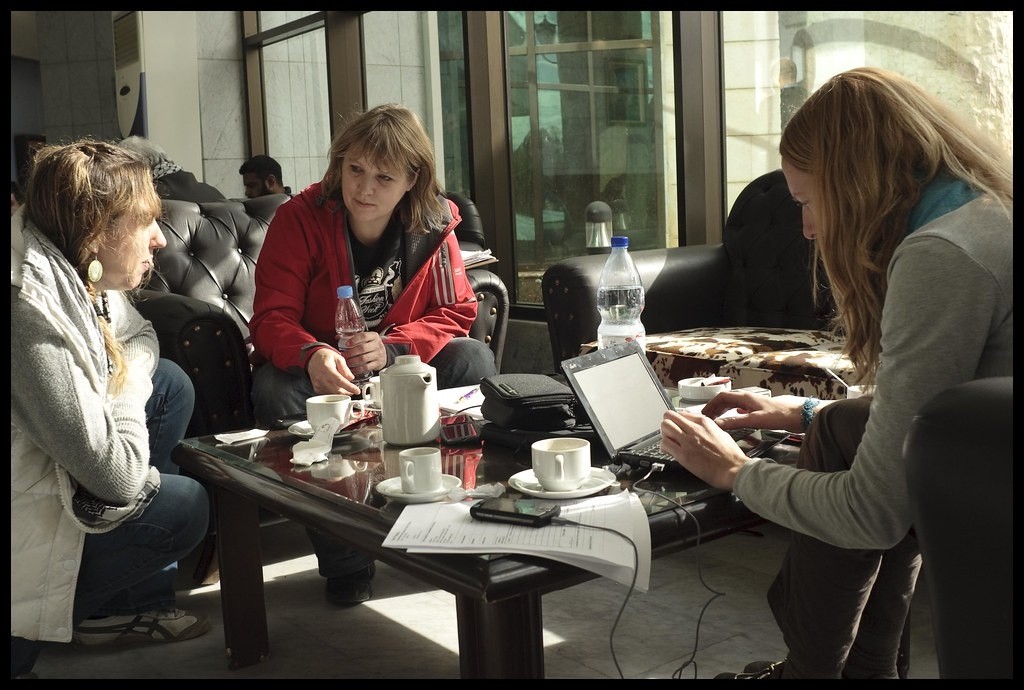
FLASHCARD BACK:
[480,366,578,431]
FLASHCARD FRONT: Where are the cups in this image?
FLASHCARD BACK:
[361,376,381,409]
[846,384,876,399]
[399,446,443,494]
[306,394,363,433]
[531,438,592,491]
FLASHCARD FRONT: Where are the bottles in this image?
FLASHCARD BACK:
[597,236,647,356]
[335,286,374,382]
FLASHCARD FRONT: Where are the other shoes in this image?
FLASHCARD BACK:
[714,658,788,680]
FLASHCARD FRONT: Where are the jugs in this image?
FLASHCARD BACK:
[379,354,441,448]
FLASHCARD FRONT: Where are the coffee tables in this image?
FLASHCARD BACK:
[172,373,802,679]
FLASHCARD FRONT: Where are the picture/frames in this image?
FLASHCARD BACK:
[603,57,648,127]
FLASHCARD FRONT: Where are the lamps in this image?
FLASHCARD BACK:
[533,16,570,64]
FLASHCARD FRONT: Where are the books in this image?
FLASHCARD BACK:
[441,384,488,420]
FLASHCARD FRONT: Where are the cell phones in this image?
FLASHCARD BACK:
[470,496,561,527]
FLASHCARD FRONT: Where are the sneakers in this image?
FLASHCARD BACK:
[71,600,211,648]
[326,556,374,604]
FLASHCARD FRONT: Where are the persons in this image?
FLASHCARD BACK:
[11,139,210,676]
[119,134,227,204]
[10,180,24,217]
[238,155,296,199]
[659,67,1013,680]
[248,105,500,606]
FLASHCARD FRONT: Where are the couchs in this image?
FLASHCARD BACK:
[127,190,509,585]
[903,373,1014,680]
[541,169,877,401]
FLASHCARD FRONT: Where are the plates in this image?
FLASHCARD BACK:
[288,420,362,439]
[375,474,463,505]
[354,399,382,412]
[508,467,616,501]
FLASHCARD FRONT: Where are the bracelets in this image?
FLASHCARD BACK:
[801,396,818,432]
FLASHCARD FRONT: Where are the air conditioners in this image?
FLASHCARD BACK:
[111,10,203,185]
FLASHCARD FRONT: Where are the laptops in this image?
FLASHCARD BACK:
[560,340,790,472]
